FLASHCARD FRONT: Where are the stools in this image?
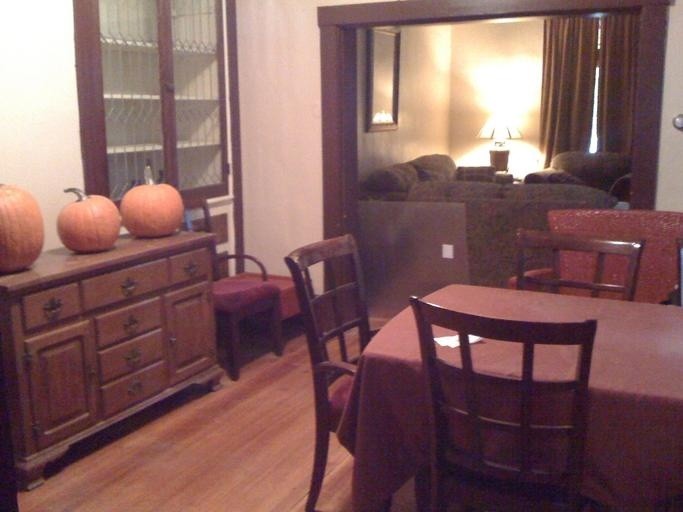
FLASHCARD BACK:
[236,268,307,324]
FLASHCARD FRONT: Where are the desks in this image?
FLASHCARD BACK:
[334,278,683,511]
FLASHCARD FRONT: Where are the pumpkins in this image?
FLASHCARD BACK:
[119,166,184,239]
[1,185,44,275]
[56,188,121,253]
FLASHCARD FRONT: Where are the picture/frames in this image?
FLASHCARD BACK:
[364,24,402,132]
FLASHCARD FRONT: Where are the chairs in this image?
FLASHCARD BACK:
[499,205,683,304]
[281,230,382,512]
[402,292,597,512]
[181,199,282,381]
[507,225,644,302]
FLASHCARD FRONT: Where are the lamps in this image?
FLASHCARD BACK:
[478,114,522,172]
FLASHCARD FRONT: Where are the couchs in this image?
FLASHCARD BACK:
[356,148,632,289]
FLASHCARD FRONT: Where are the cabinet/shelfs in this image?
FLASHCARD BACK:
[1,233,219,491]
[72,0,229,209]
